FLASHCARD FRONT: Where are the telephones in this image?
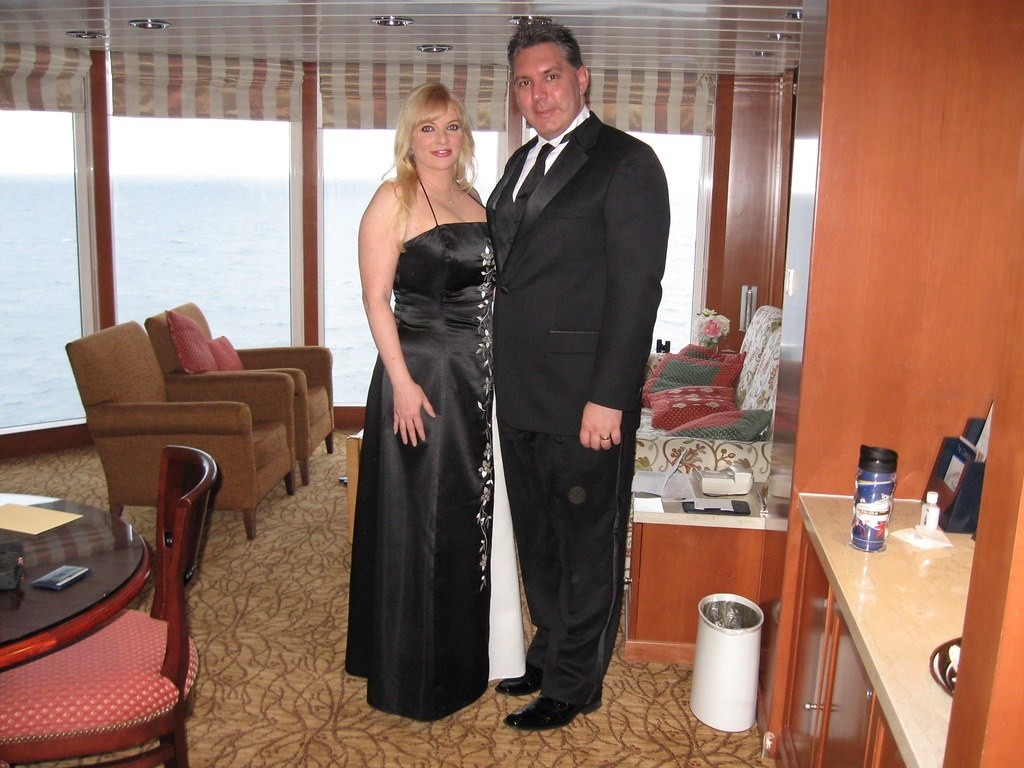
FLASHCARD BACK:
[699,463,753,497]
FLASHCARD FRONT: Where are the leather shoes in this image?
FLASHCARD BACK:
[503,689,603,731]
[495,675,543,698]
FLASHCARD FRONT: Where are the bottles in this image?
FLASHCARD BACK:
[850,444,898,554]
[920,492,941,532]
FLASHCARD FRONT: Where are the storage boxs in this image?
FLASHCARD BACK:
[920,417,987,534]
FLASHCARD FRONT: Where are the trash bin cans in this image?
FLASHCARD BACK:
[687,594,763,733]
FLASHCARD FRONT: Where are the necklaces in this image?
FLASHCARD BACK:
[420,177,453,204]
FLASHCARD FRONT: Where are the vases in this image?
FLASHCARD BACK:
[699,332,718,352]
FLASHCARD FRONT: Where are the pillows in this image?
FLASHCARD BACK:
[647,386,739,430]
[662,409,773,442]
[164,309,219,375]
[643,353,744,409]
[677,343,746,365]
[208,335,245,371]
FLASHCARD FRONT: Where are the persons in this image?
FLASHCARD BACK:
[344,80,496,721]
[484,19,671,731]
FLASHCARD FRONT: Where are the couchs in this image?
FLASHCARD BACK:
[623,306,783,589]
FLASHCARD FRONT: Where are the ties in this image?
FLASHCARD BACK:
[515,116,590,209]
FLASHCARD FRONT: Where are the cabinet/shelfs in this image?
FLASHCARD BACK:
[775,521,907,768]
[626,516,766,644]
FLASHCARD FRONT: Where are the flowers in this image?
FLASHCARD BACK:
[694,308,730,343]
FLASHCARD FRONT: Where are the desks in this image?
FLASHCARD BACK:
[0,492,158,669]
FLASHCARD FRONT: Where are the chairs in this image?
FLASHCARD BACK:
[65,320,297,541]
[0,444,218,768]
[144,302,334,484]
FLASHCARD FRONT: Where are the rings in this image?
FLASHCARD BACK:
[601,437,610,440]
